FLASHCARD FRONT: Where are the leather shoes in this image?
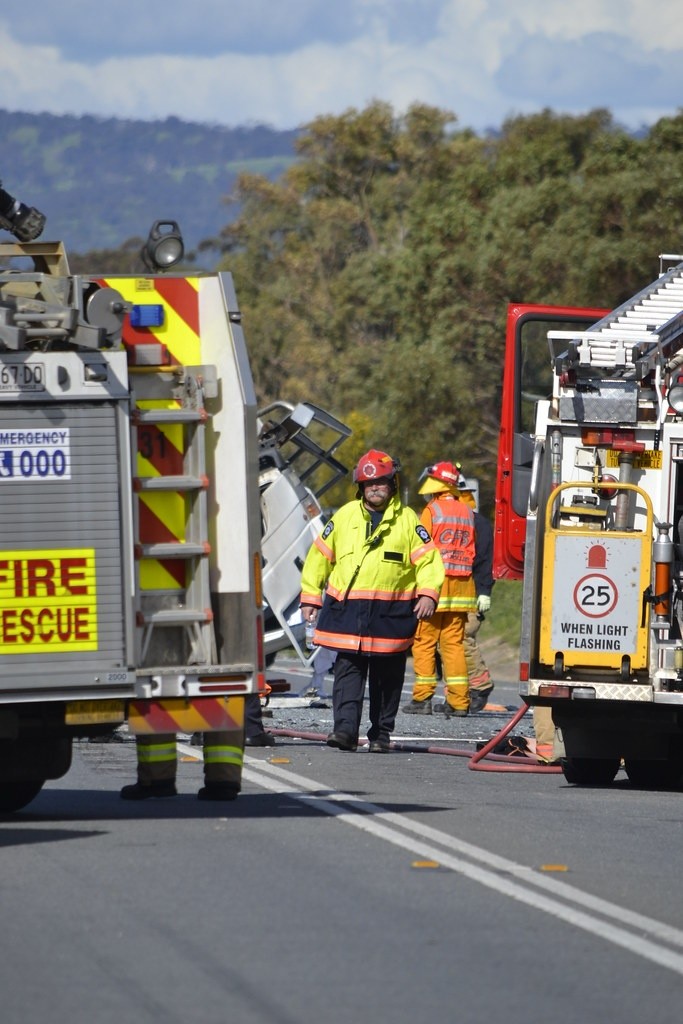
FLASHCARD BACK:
[434,702,467,717]
[469,682,494,714]
[327,732,358,751]
[369,740,389,753]
[402,700,432,715]
[88,732,124,743]
[244,733,275,746]
[190,731,203,745]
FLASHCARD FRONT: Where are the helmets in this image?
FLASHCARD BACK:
[456,473,477,492]
[352,449,396,484]
[426,461,461,485]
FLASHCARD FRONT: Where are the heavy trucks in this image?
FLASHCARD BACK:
[257,394,352,658]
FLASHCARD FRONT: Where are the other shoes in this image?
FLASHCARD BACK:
[197,781,238,801]
[120,779,177,800]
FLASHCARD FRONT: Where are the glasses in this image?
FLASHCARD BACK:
[365,479,387,486]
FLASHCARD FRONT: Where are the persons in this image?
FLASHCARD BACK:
[530,706,566,768]
[400,463,495,717]
[302,647,338,697]
[300,451,446,755]
[88,693,275,805]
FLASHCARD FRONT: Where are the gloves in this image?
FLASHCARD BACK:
[477,594,491,613]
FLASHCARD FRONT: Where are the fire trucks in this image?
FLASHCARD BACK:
[0,184,267,817]
[489,254,681,790]
